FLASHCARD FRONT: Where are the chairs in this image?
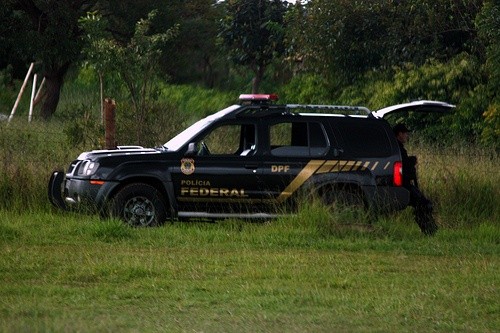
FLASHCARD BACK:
[239,144,256,156]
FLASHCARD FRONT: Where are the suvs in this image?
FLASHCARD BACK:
[47,95,460,236]
